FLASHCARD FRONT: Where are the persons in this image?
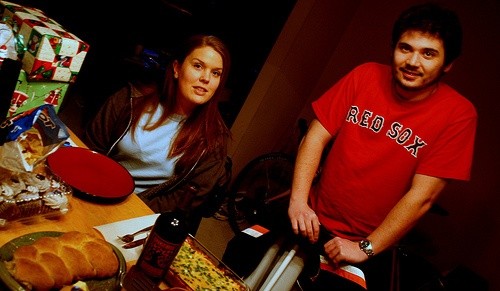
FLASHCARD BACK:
[82,35,233,214]
[218,3,477,291]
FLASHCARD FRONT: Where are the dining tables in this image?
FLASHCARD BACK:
[0,121,169,290]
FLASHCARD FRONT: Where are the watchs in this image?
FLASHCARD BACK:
[359,239,374,259]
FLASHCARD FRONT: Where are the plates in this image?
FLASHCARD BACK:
[168,233,251,291]
[0,231,127,291]
[47,146,135,204]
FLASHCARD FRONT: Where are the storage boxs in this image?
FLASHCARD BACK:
[0,0,90,119]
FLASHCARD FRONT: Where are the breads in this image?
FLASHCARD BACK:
[5,231,119,291]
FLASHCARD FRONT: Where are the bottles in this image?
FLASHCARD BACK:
[136,186,200,279]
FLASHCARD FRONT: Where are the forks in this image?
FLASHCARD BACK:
[117,225,153,243]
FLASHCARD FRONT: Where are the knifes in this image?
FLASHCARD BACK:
[122,237,147,249]
